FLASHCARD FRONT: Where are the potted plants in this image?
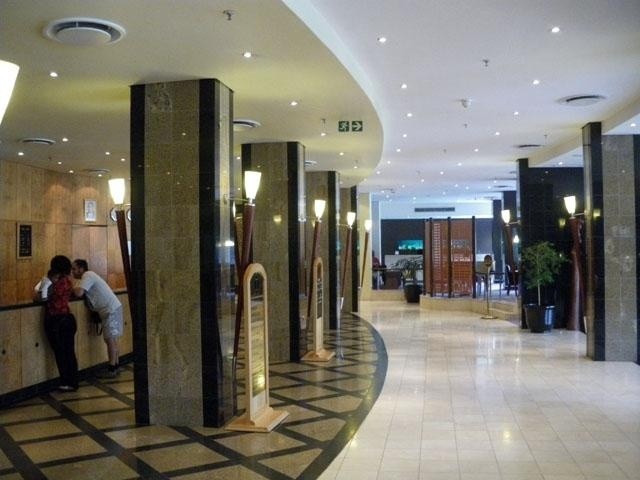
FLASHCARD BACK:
[387,255,424,303]
[518,240,574,333]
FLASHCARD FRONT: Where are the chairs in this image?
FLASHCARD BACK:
[505,264,519,296]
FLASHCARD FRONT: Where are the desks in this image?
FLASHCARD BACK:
[476,271,504,297]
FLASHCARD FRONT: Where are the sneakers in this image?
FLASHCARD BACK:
[115,363,120,375]
[94,364,116,378]
[56,381,79,392]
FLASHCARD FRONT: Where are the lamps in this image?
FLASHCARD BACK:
[107,177,131,318]
[301,200,326,330]
[563,195,588,334]
[501,209,519,298]
[336,211,356,310]
[222,170,262,381]
[357,220,372,290]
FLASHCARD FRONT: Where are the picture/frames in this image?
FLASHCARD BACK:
[84,199,97,222]
[16,222,33,260]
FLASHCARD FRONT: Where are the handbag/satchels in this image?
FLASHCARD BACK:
[84,293,103,335]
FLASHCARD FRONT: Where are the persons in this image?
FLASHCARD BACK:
[32,254,82,394]
[372,251,379,267]
[33,269,60,301]
[72,259,125,380]
